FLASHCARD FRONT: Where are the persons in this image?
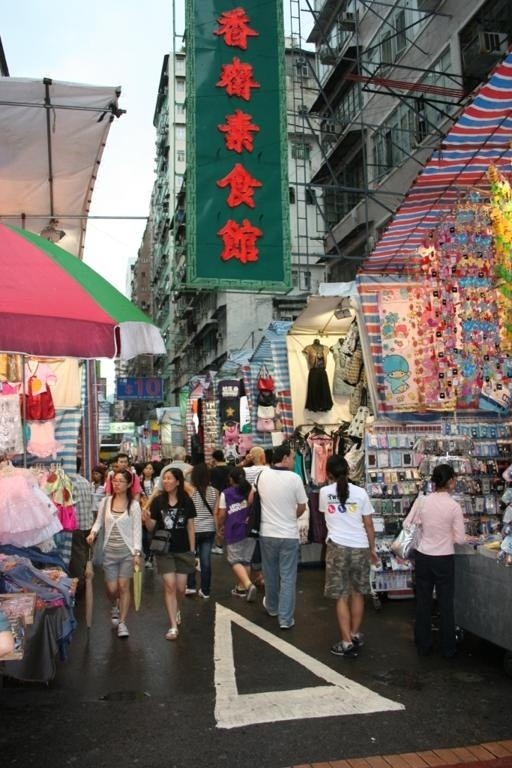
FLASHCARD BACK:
[68,446,309,640]
[319,455,377,658]
[404,464,480,657]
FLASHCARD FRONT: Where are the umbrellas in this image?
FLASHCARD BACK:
[0,217,168,361]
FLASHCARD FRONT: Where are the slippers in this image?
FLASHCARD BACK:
[166,610,182,640]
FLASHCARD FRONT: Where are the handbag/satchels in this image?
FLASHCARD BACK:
[133,566,141,612]
[149,530,172,554]
[245,493,260,538]
[256,364,275,432]
[390,521,422,560]
[339,323,370,483]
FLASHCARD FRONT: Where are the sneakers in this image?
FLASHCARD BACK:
[117,621,129,637]
[144,557,153,567]
[232,572,264,603]
[280,618,295,629]
[263,596,278,616]
[110,598,119,624]
[330,631,368,657]
[211,545,224,555]
[184,585,212,599]
